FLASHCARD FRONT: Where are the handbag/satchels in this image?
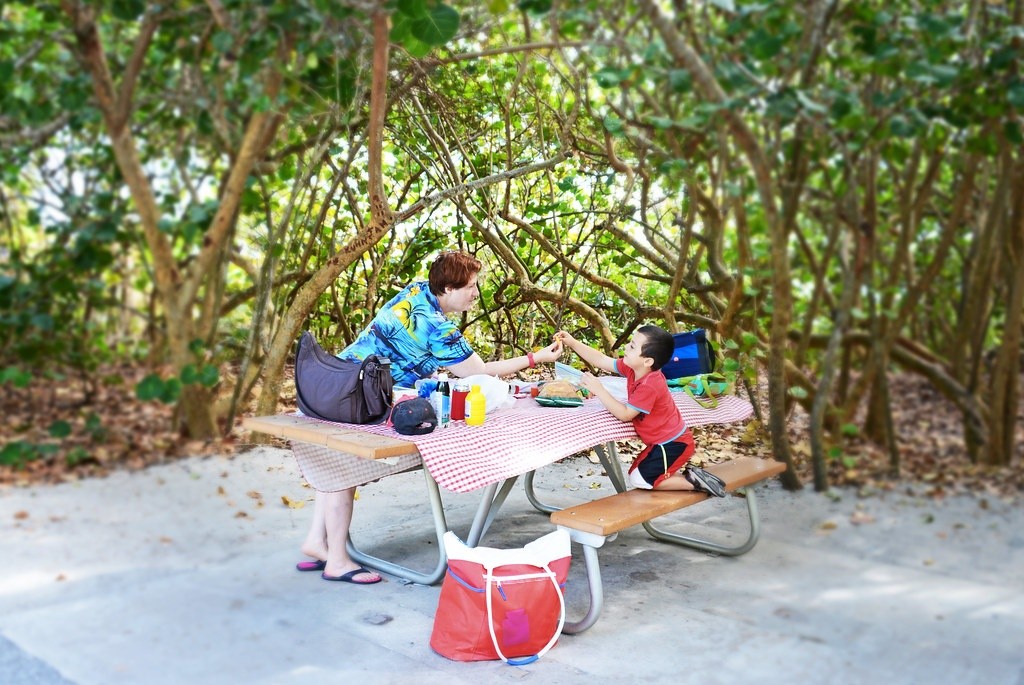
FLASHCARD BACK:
[662,328,715,380]
[294,330,393,425]
[429,527,574,665]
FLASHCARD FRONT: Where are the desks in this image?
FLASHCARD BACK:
[242,382,754,586]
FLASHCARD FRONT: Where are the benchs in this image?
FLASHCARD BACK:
[549,453,786,637]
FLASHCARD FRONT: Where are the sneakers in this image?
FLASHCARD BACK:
[682,462,726,498]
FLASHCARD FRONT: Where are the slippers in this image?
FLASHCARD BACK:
[296,559,326,571]
[322,565,380,583]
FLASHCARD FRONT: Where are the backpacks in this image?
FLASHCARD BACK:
[666,371,728,408]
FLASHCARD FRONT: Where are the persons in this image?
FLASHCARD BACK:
[301,248,565,585]
[552,324,727,498]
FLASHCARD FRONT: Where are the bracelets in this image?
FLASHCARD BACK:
[527,351,535,367]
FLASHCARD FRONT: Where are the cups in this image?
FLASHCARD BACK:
[451,383,470,420]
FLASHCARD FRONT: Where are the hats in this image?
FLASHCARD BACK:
[390,398,438,435]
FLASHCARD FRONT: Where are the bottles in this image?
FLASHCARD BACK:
[436,373,451,428]
[465,385,486,427]
